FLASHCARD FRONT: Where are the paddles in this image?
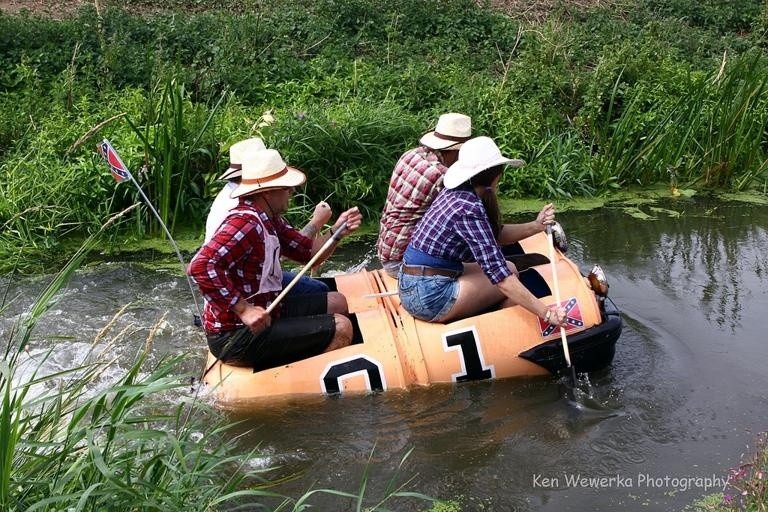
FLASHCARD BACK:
[549,221,589,402]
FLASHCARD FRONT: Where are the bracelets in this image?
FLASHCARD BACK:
[544,307,552,324]
[329,227,341,243]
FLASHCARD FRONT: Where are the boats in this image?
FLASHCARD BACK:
[204,220,623,399]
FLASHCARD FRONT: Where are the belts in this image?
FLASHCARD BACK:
[205,331,222,337]
[402,264,462,278]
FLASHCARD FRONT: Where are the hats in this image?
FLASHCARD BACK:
[218,137,307,199]
[420,113,524,189]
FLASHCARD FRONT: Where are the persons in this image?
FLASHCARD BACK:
[396,137,571,330]
[188,148,364,368]
[374,111,473,279]
[202,136,335,310]
